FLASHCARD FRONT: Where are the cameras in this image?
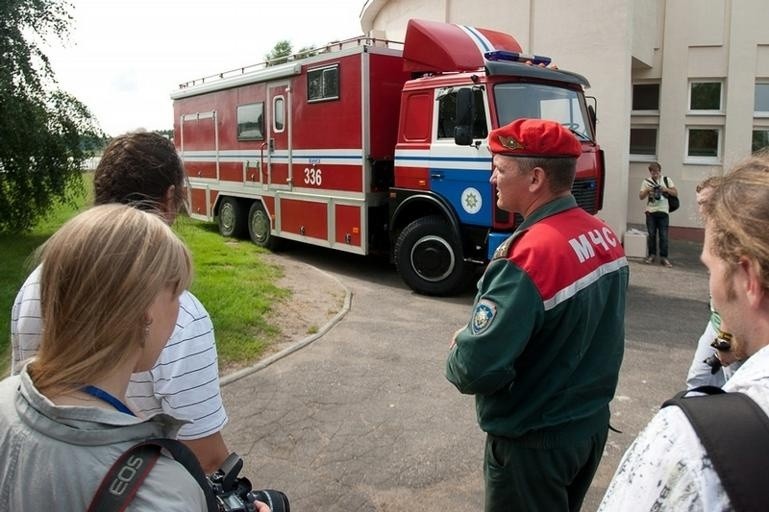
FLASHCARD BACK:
[208,452,291,512]
[653,185,661,200]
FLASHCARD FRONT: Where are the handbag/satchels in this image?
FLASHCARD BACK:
[662,175,680,212]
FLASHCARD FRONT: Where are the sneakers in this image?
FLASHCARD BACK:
[647,253,673,268]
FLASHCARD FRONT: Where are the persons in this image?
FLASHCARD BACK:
[445,116,629,512]
[596,145,769,512]
[1,129,230,472]
[639,162,678,268]
[1,204,271,512]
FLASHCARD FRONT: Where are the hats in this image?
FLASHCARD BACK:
[487,117,583,157]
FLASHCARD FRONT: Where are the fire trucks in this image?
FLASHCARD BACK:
[170,18,606,297]
